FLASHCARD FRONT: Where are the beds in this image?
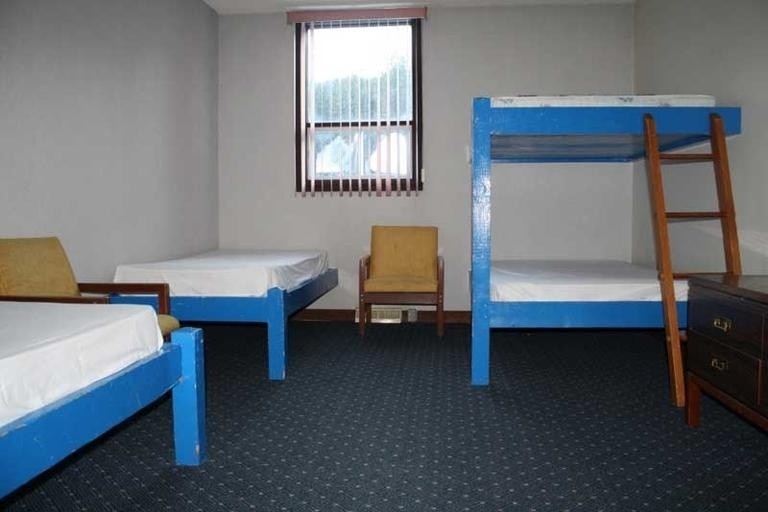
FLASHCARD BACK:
[115,251,337,382]
[0,301,207,500]
[472,95,741,388]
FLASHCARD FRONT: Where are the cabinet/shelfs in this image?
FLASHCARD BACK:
[686,275,768,431]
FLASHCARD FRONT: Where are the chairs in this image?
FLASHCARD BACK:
[0,237,180,335]
[357,225,445,337]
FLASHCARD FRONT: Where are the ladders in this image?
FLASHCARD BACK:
[642,114,740,408]
